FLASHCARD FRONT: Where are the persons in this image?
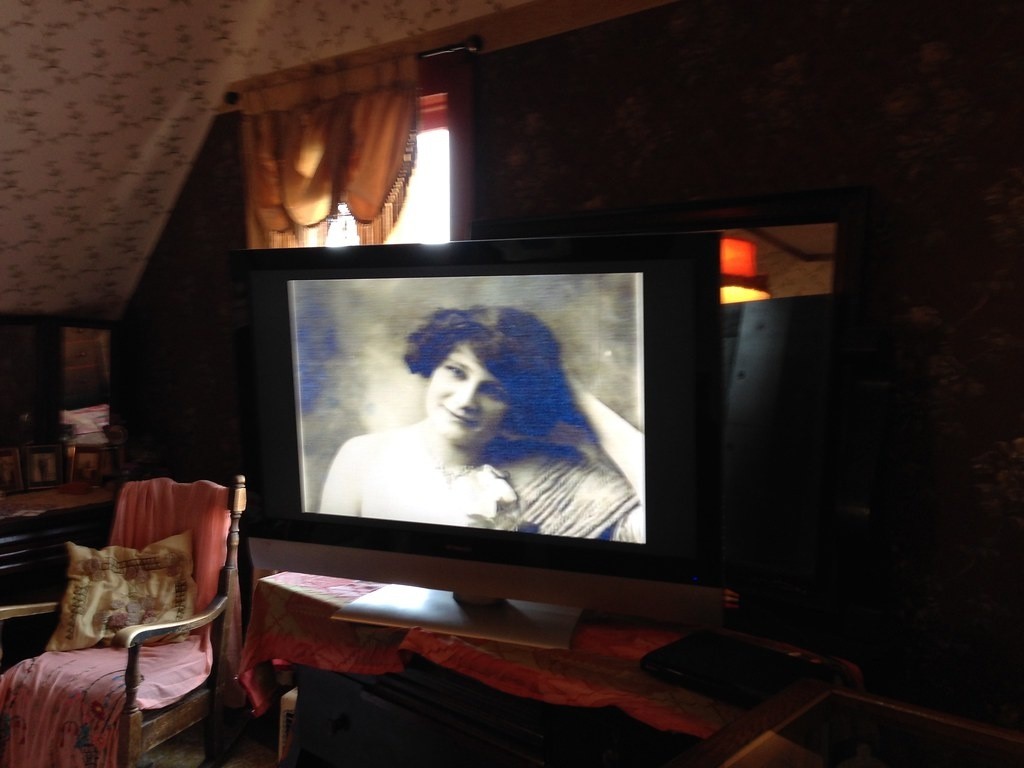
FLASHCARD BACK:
[319,304,642,543]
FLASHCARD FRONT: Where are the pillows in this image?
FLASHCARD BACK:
[43,526,198,654]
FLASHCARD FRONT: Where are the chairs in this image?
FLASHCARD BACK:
[0,470,254,768]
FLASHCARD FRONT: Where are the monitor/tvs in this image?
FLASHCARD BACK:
[229,231,724,651]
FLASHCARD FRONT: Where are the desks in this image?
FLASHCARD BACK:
[0,478,132,603]
[232,568,871,768]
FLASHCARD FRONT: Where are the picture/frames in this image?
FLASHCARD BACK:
[0,441,108,495]
